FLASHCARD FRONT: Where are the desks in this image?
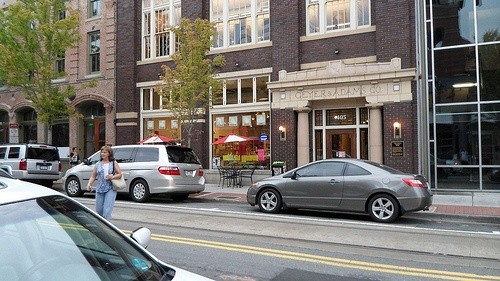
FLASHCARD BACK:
[225,167,244,186]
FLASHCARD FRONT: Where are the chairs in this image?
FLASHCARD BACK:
[217,166,255,189]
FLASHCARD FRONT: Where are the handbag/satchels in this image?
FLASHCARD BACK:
[111,173,127,191]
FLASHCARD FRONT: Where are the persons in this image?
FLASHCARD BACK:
[87,146,122,222]
[70,147,82,167]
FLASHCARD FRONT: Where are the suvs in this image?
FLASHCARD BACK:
[62,144,206,203]
[0,143,62,189]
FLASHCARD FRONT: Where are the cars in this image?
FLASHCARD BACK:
[0,164,216,281]
[430,153,463,178]
[246,156,434,223]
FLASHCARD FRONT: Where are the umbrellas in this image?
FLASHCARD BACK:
[136,134,176,143]
[209,132,258,155]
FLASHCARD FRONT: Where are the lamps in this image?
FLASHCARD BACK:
[393,122,401,139]
[279,126,287,141]
